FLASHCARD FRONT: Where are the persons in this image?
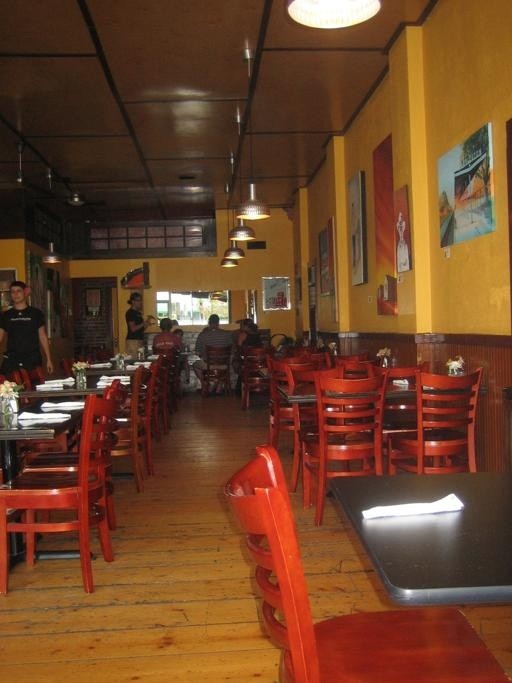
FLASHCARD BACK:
[125,293,151,360]
[0,281,53,404]
[152,313,261,395]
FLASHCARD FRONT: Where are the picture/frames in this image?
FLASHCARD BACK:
[324,468,511,609]
[261,275,291,312]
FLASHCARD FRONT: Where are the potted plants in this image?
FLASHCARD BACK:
[261,275,291,312]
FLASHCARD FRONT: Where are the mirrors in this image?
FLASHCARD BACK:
[155,288,231,327]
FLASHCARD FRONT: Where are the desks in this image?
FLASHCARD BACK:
[324,468,511,609]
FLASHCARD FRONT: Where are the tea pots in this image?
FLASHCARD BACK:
[155,288,231,327]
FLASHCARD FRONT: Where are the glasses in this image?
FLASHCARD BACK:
[235,46,273,221]
[227,106,258,243]
[220,181,241,268]
[12,140,28,183]
[224,151,247,260]
[67,189,88,208]
[286,0,382,32]
[41,166,62,265]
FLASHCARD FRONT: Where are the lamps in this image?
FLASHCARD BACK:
[223,444,512,683]
[41,166,62,265]
[286,0,382,32]
[67,189,88,208]
[220,181,241,268]
[12,140,28,183]
[224,151,247,260]
[235,46,273,221]
[227,106,258,243]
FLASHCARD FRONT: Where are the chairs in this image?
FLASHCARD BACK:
[191,342,273,413]
[265,343,485,527]
[0,342,190,531]
[0,380,119,594]
[223,444,512,683]
